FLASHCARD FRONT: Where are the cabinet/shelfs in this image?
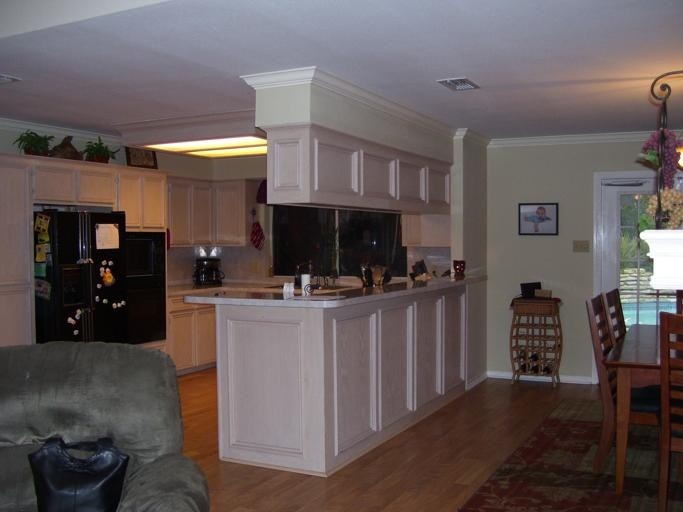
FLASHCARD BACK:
[166,176,211,247]
[114,164,167,232]
[1,155,37,347]
[32,156,114,207]
[212,179,260,249]
[168,295,217,375]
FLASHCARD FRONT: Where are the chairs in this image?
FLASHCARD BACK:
[604,288,629,348]
[660,312,683,512]
[1,343,211,512]
[584,293,662,470]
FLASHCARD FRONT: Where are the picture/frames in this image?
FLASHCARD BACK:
[126,146,159,167]
[518,203,558,235]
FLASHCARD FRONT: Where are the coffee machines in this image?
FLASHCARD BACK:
[192,257,225,288]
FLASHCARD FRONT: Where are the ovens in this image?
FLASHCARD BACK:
[125,231,167,344]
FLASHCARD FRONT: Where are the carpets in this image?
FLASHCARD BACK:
[455,398,682,511]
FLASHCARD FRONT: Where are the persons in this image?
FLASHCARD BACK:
[525,207,552,232]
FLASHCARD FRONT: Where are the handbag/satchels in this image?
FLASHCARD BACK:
[28,433,130,512]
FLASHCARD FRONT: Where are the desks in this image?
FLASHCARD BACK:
[508,298,564,388]
[602,324,682,491]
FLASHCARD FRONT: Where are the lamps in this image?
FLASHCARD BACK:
[640,68,683,314]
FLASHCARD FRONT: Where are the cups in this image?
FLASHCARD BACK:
[454,260,465,273]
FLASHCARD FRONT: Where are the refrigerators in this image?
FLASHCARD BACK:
[33,210,126,343]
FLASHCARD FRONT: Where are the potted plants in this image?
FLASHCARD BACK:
[83,136,120,163]
[13,129,56,155]
